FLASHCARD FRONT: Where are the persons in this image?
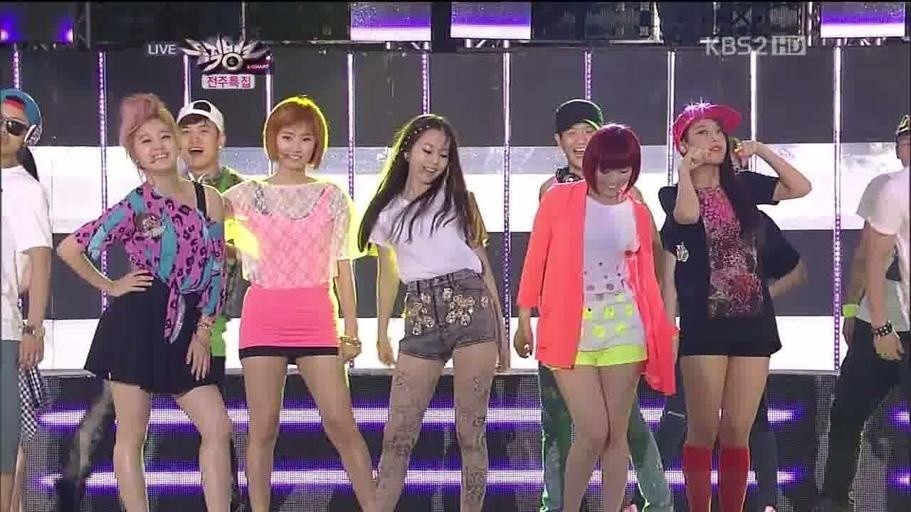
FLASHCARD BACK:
[864,164,911,365]
[11,148,46,510]
[539,100,671,512]
[358,114,510,512]
[220,96,375,512]
[514,123,661,512]
[659,136,810,510]
[818,114,911,512]
[679,103,813,507]
[1,88,52,512]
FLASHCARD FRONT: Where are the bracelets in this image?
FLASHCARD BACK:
[870,319,893,338]
[340,337,359,347]
[200,321,212,336]
[843,304,858,320]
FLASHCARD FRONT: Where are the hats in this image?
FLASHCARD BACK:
[176,99,224,133]
[0,88,41,125]
[672,102,742,151]
[894,114,911,136]
[553,99,603,131]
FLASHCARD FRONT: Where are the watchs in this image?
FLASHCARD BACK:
[23,324,47,340]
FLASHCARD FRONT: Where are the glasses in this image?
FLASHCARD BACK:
[0,113,29,137]
